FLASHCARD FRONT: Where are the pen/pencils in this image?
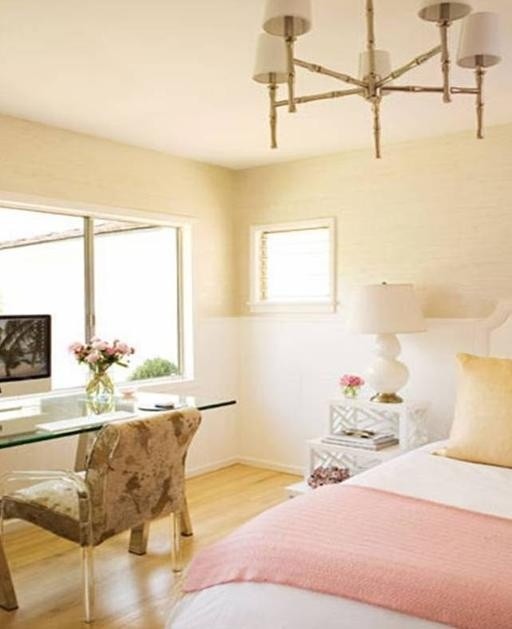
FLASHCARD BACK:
[155,405,174,409]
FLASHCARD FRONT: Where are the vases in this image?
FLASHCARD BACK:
[345,385,356,397]
[83,373,115,394]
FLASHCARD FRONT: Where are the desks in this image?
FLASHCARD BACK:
[1,385,237,612]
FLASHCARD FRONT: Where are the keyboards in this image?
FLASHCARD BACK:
[36,410,136,434]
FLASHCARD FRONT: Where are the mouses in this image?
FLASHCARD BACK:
[155,402,174,408]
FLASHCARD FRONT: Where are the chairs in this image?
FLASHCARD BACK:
[0,407,202,625]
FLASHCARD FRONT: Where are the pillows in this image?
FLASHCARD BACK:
[432,350,512,470]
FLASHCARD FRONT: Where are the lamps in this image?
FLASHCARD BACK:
[337,280,424,398]
[247,0,509,161]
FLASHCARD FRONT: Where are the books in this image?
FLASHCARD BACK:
[327,429,397,444]
[322,437,399,450]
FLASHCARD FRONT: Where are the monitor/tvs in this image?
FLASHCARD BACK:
[1,314,53,412]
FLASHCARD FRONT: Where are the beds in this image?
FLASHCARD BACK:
[165,304,512,629]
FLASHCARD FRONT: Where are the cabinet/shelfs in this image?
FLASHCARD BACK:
[284,394,430,498]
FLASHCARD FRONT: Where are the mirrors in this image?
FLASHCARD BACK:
[249,217,337,313]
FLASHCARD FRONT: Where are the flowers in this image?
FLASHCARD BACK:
[339,375,363,389]
[68,336,136,378]
